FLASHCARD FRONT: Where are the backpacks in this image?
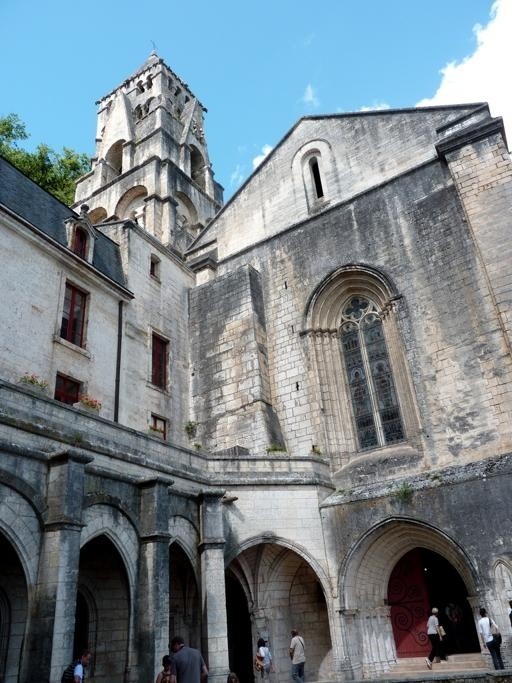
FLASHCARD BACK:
[160,671,173,683]
[61,662,81,683]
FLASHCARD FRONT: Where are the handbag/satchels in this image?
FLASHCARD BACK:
[492,633,502,644]
[254,652,263,671]
[440,626,446,636]
[270,664,276,674]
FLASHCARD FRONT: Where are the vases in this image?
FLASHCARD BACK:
[73,402,100,415]
[17,382,48,395]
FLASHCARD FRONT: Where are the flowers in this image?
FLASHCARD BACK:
[18,371,49,391]
[80,394,101,410]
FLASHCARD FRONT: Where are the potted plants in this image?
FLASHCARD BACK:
[143,428,164,439]
[267,447,287,455]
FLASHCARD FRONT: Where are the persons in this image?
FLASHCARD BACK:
[258,637,271,683]
[156,635,208,683]
[61,651,91,683]
[227,672,239,683]
[289,630,307,683]
[425,608,443,670]
[476,609,505,670]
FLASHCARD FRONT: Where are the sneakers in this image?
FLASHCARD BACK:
[425,659,432,670]
